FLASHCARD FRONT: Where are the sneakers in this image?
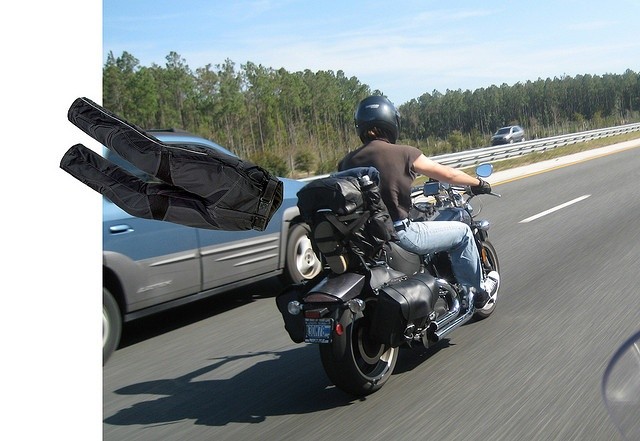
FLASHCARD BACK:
[472,270,501,311]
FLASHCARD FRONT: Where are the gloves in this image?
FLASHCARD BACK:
[471,178,491,195]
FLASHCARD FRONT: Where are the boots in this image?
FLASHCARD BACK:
[313,220,391,275]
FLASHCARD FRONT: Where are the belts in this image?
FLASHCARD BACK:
[394,221,410,232]
[253,174,279,232]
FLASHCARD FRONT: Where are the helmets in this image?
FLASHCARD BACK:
[354,96,402,144]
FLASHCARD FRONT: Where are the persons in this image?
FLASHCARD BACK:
[337,96,500,310]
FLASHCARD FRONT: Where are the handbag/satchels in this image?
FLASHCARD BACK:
[295,166,396,267]
[377,271,440,352]
[274,270,326,343]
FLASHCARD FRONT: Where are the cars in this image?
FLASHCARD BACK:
[492,125,526,144]
[104,129,324,365]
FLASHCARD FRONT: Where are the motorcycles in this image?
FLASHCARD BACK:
[287,162,501,398]
